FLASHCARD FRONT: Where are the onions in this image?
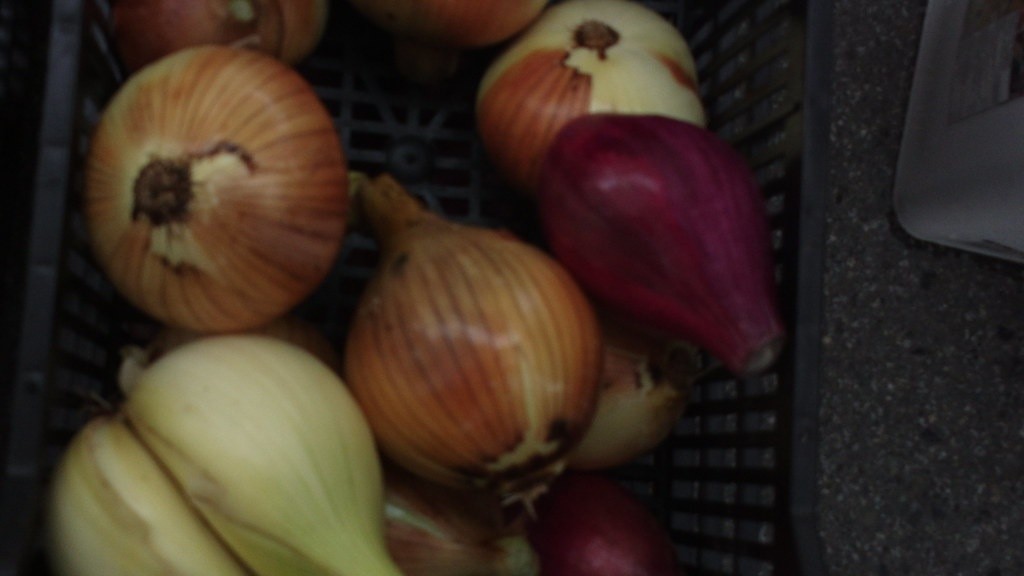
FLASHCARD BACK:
[40,0,784,576]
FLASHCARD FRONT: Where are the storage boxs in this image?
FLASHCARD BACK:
[2,0,829,576]
[890,1,1024,267]
